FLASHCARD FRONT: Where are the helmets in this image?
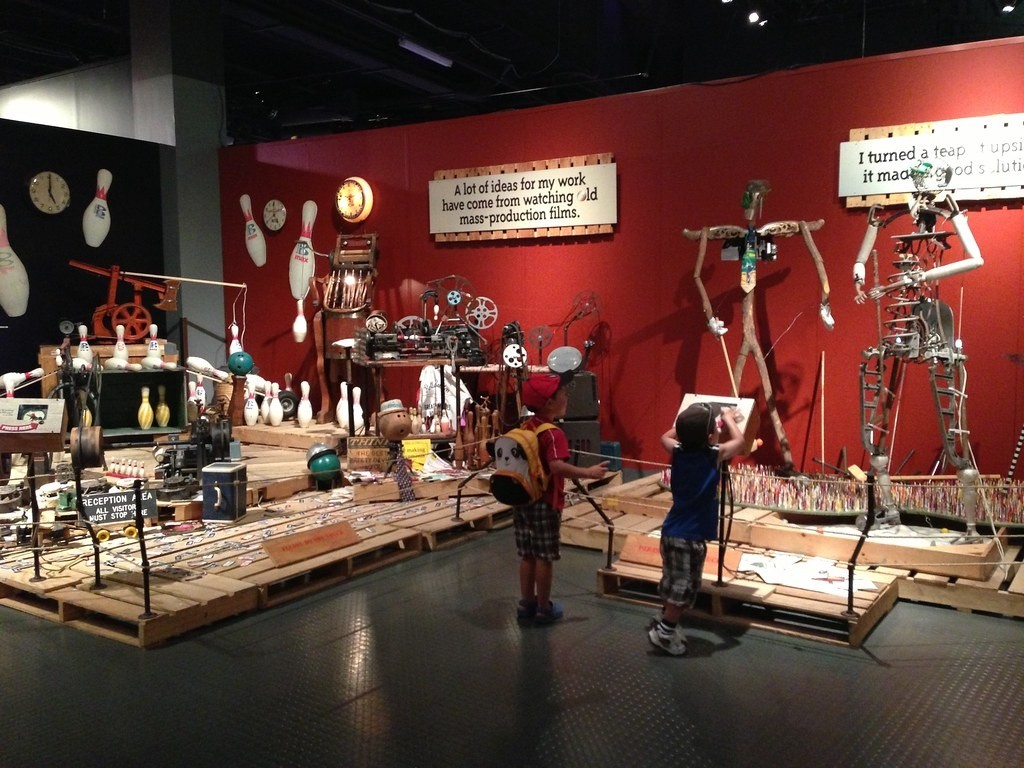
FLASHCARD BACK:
[306,444,336,469]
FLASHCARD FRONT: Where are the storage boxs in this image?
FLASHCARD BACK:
[201,462,248,526]
[38,344,180,399]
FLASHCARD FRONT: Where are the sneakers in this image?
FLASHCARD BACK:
[649,625,686,656]
[650,614,688,644]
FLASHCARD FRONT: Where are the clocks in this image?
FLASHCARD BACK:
[263,199,287,232]
[28,170,71,215]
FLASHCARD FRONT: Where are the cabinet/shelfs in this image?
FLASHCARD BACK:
[354,358,470,444]
[56,367,188,440]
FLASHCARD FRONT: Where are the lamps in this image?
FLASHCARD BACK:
[335,176,374,223]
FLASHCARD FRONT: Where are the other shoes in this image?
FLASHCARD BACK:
[535,600,562,624]
[517,595,537,619]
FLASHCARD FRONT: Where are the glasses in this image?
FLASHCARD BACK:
[688,403,712,437]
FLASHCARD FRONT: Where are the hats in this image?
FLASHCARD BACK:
[376,399,408,417]
[522,369,574,409]
[675,402,721,442]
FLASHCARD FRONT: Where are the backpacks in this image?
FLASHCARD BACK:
[488,423,562,508]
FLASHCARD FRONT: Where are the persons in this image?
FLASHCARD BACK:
[509,369,611,625]
[646,402,746,655]
